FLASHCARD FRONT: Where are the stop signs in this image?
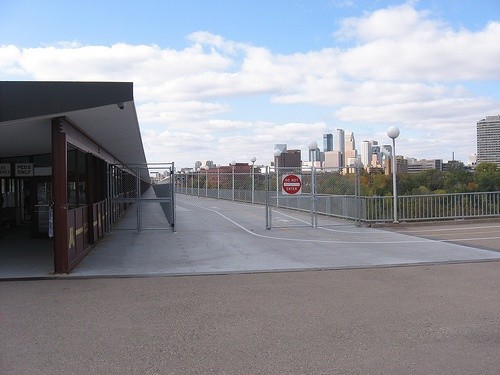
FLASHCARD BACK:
[281,174,302,196]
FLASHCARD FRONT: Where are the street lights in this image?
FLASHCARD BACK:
[190,169,194,196]
[204,166,209,198]
[274,149,281,207]
[250,156,257,204]
[196,168,201,198]
[185,170,189,195]
[307,139,318,213]
[387,125,400,224]
[230,160,236,201]
[216,163,221,199]
[180,171,184,193]
